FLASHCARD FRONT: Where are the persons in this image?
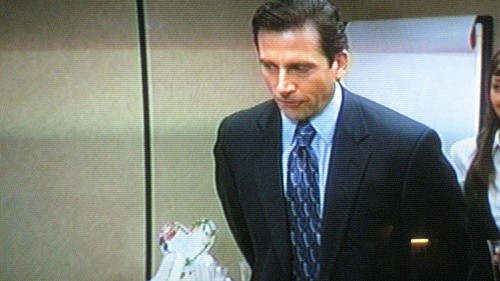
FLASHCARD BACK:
[449,55,500,281]
[214,0,476,281]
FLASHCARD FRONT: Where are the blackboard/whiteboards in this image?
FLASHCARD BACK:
[336,14,494,196]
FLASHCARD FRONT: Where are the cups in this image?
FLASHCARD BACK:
[487,240,500,281]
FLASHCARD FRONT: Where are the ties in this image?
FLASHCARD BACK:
[286,119,321,281]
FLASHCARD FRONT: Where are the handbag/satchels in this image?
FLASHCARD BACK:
[150,217,233,281]
